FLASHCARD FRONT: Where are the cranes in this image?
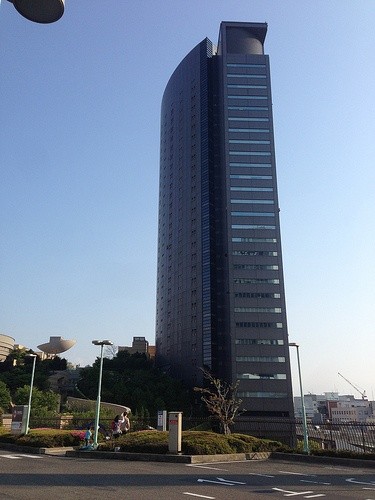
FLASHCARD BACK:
[337,372,367,399]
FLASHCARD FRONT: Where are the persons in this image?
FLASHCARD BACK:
[112,414,124,438]
[85,427,92,447]
[121,412,130,435]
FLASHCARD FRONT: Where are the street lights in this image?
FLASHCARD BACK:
[23,353,38,436]
[90,338,114,450]
[288,342,311,456]
